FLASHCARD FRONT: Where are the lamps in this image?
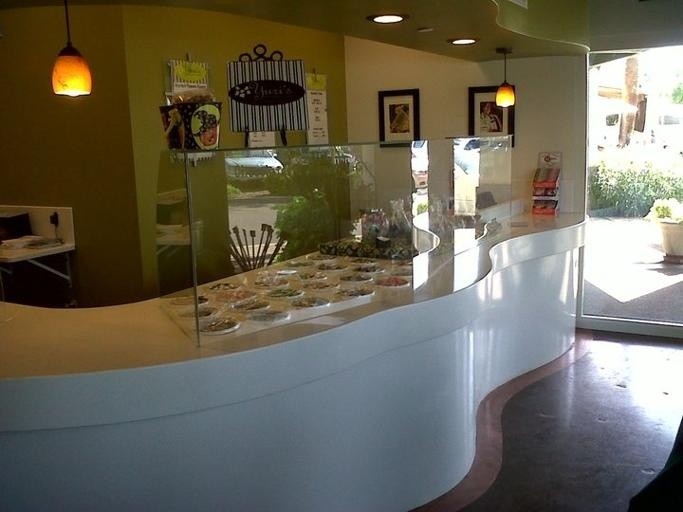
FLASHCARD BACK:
[51,0,92,96]
[494,48,516,107]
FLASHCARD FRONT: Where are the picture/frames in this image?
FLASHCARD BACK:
[468,85,515,149]
[377,88,421,147]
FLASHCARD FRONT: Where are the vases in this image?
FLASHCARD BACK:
[659,220,683,257]
